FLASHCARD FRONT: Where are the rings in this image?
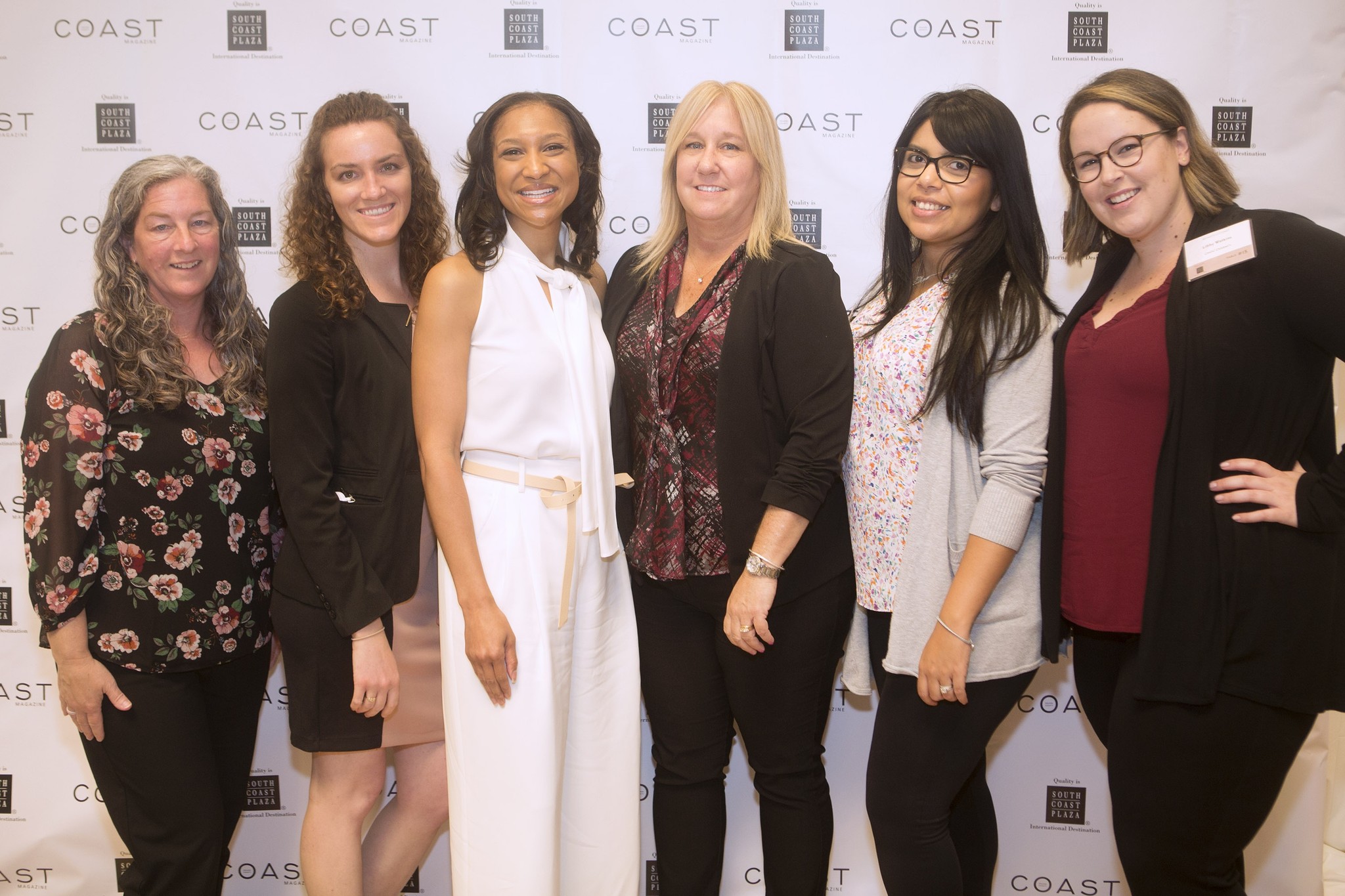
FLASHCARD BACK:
[940,685,953,694]
[740,625,754,633]
[365,696,376,702]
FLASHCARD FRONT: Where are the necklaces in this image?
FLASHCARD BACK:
[687,248,731,283]
[911,256,961,286]
[412,319,417,325]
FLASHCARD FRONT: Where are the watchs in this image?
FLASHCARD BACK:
[746,554,781,578]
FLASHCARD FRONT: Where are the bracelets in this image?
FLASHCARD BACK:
[747,548,785,571]
[936,615,975,649]
[350,626,385,641]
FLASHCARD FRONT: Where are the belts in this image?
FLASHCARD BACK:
[462,460,635,630]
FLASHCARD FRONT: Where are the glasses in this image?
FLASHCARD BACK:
[1066,129,1170,183]
[896,147,992,184]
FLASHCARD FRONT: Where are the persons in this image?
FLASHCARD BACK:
[410,91,607,896]
[602,80,856,896]
[1040,68,1345,896]
[841,89,1058,896]
[264,92,453,896]
[19,155,279,896]
